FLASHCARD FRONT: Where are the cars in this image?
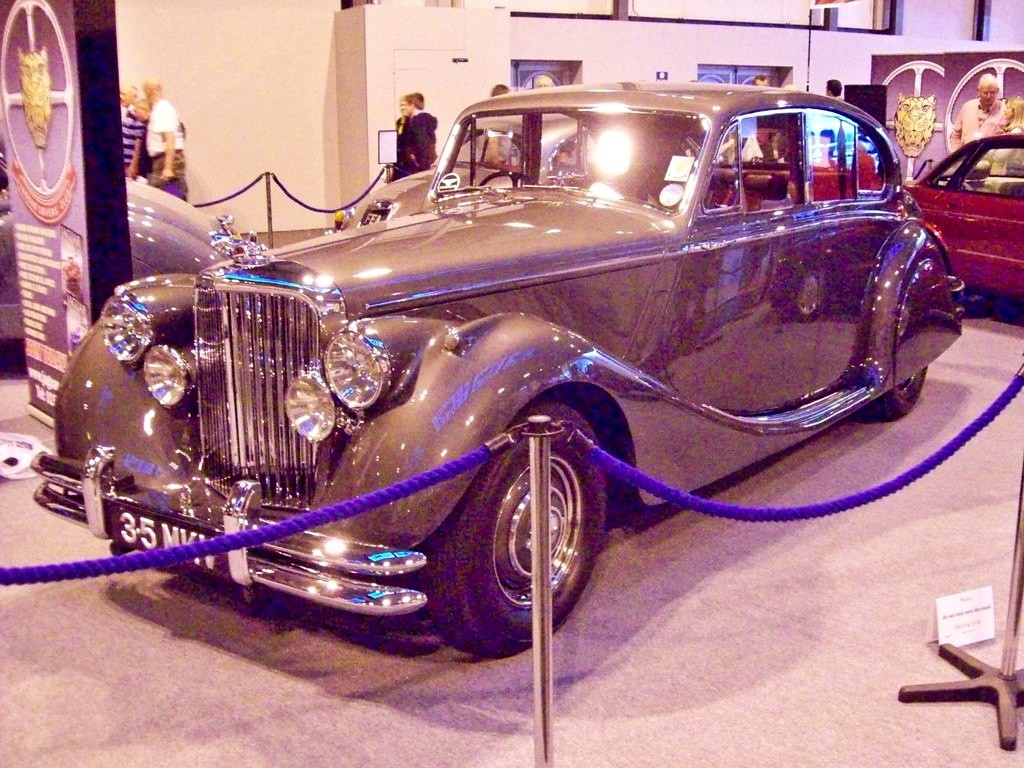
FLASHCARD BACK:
[0,178,267,345]
[901,134,1024,307]
[31,82,967,658]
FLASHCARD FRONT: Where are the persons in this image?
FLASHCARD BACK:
[119,79,189,204]
[537,76,551,88]
[754,74,770,86]
[826,79,844,101]
[492,84,510,97]
[949,73,1007,153]
[392,93,438,182]
[989,96,1024,175]
